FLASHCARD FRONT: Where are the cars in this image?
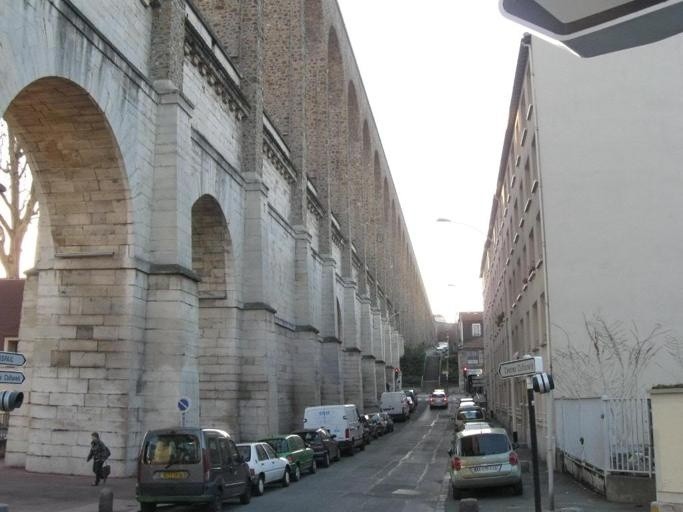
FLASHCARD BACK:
[235,442,291,496]
[429,389,448,409]
[359,412,394,444]
[454,397,494,431]
[448,427,523,500]
[401,390,417,411]
[289,427,340,467]
[259,434,317,481]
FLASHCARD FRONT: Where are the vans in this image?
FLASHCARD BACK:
[136,427,252,512]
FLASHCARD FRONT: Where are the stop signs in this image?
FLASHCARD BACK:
[177,397,190,413]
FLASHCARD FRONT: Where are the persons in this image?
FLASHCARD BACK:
[86,431,111,485]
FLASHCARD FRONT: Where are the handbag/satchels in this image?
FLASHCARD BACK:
[97,464,111,479]
[98,447,110,462]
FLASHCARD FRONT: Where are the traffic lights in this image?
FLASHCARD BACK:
[463,367,467,379]
[394,367,400,381]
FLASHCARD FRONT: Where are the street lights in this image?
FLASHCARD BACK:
[438,218,518,442]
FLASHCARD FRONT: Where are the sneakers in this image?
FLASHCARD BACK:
[91,480,100,487]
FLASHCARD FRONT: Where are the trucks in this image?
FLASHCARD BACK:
[302,404,364,457]
[379,392,410,422]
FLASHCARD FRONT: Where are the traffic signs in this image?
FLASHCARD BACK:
[498,356,542,379]
[0,351,26,366]
[0,371,25,384]
